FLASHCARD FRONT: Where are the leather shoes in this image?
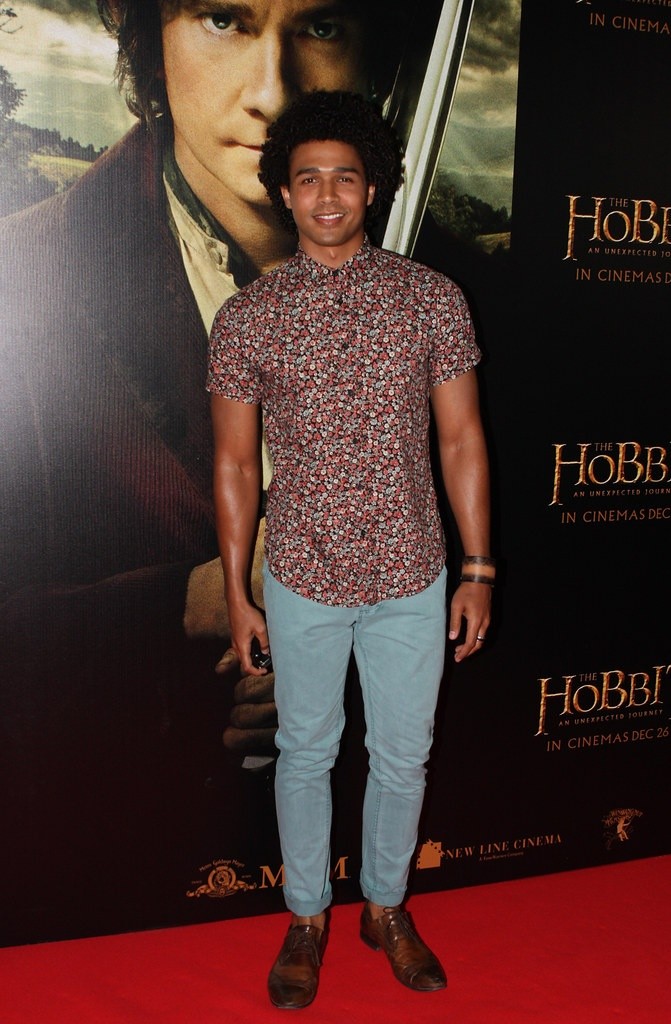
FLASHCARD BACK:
[360,901,447,991]
[267,924,328,1008]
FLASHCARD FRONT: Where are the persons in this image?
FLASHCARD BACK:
[204,88,491,1010]
[0,0,408,947]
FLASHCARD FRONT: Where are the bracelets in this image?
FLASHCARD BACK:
[460,556,496,588]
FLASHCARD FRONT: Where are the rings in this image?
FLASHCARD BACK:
[477,636,484,641]
